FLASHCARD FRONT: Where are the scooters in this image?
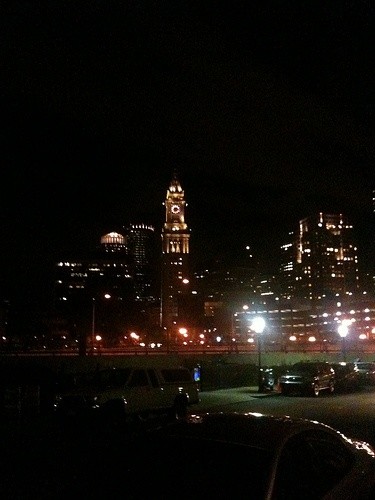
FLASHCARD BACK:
[259,367,275,393]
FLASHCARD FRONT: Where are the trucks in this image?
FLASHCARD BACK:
[47,364,201,429]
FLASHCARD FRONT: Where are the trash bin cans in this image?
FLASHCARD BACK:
[257,368,274,392]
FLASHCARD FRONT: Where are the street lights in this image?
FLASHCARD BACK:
[337,325,349,362]
[251,318,265,383]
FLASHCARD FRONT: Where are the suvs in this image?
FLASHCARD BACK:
[278,362,337,397]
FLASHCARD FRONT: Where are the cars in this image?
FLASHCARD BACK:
[332,359,375,395]
[59,411,375,500]
